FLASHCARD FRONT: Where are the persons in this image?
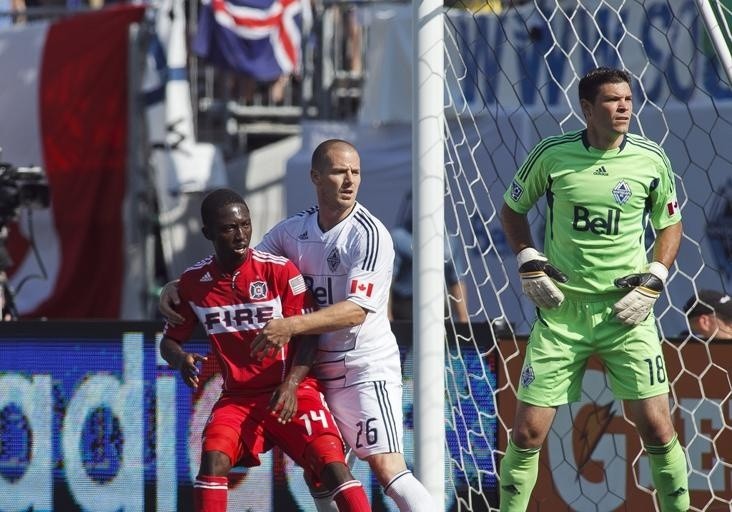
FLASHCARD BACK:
[679,289,732,340]
[389,188,470,323]
[160,140,440,512]
[159,187,371,512]
[497,68,691,512]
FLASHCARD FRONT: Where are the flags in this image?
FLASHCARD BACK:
[190,0,317,83]
[0,3,145,319]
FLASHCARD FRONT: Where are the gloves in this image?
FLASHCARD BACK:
[514,247,568,308]
[611,262,668,328]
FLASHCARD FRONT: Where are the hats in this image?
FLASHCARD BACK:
[685,288,732,319]
